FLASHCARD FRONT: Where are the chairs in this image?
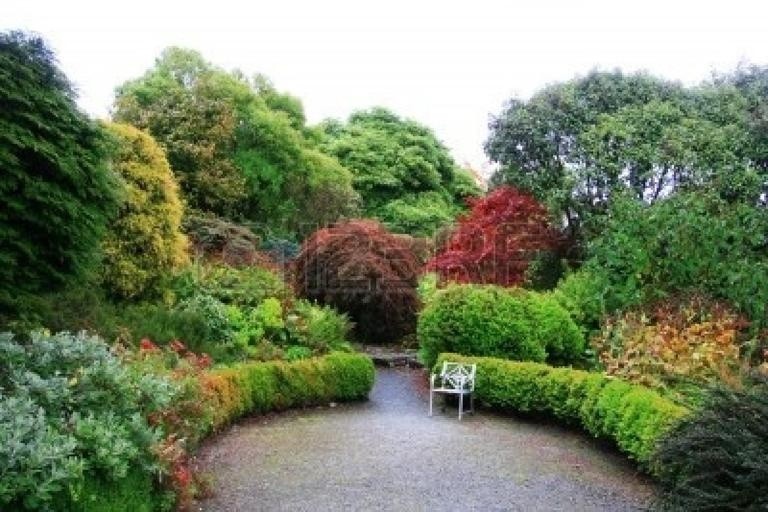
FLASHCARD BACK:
[428,360,476,421]
